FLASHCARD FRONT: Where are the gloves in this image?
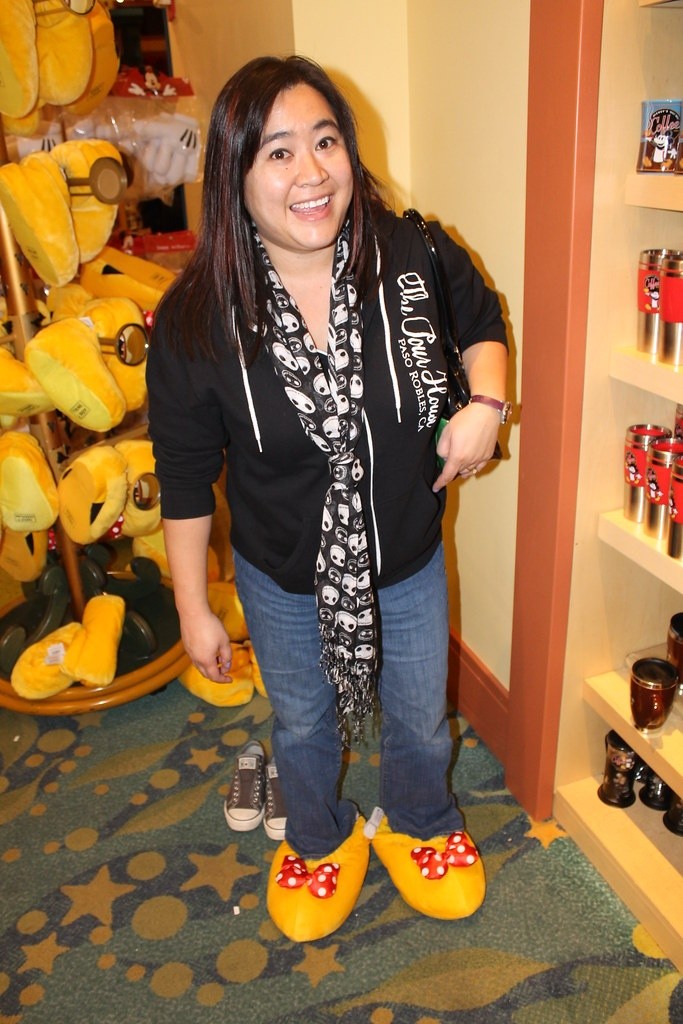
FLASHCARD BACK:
[17,112,202,186]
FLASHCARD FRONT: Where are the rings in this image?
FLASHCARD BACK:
[473,467,478,475]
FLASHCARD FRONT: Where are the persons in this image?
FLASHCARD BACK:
[147,56,511,940]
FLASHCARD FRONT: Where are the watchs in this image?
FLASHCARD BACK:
[469,394,511,425]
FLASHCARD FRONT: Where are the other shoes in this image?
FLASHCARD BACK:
[0,0,119,136]
[266,812,486,943]
[0,138,271,707]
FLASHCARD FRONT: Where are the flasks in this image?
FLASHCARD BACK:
[624,403,683,559]
[637,249,683,366]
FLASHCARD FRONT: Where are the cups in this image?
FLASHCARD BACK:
[598,730,683,836]
[629,612,683,730]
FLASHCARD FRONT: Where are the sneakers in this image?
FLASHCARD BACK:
[224,739,287,840]
